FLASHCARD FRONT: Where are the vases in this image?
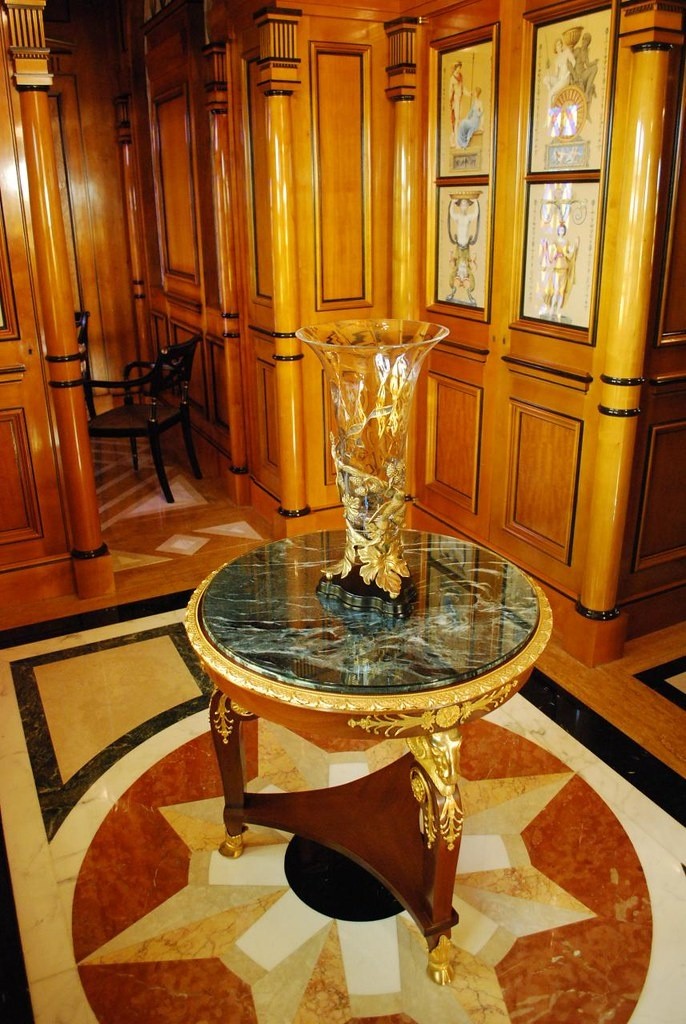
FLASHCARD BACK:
[293,315,453,622]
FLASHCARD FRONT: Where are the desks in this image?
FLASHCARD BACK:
[181,527,554,987]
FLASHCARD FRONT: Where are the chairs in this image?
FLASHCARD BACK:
[83,333,203,503]
[75,311,97,421]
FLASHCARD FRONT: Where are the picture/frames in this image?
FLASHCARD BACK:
[518,172,601,333]
[434,183,489,312]
[525,3,612,175]
[435,36,492,180]
